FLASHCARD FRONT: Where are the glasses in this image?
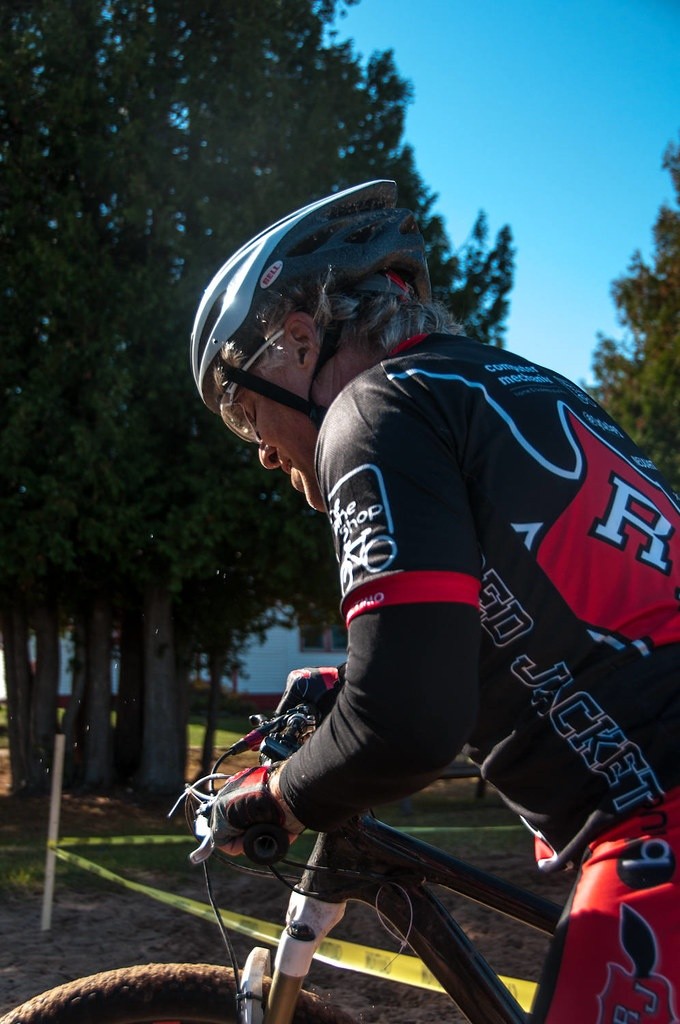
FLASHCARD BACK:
[220,330,284,444]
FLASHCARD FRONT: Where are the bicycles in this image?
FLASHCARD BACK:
[0,704,566,1024]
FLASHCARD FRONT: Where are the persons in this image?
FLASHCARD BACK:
[193,175,680,1023]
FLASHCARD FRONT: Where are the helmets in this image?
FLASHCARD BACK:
[190,179,432,416]
